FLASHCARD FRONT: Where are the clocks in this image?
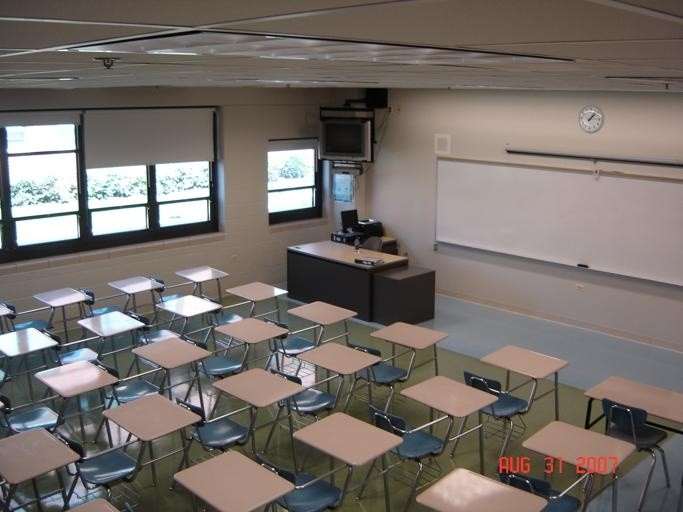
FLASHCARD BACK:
[576,104,604,134]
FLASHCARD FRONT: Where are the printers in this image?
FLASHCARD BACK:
[352,218,383,239]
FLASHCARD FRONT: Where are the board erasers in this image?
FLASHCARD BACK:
[577,263,589,269]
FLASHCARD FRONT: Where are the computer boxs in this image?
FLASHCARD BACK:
[331,231,364,245]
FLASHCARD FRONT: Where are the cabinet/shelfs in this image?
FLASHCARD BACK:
[373,264,435,325]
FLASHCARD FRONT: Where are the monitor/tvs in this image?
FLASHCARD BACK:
[318,120,372,162]
[341,210,358,233]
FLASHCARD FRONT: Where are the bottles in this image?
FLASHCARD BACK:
[354,237,359,255]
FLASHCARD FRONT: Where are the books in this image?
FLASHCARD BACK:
[354,256,382,266]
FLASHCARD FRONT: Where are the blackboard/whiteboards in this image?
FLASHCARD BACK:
[434,155,683,289]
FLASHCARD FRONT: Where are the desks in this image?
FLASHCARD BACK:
[1,264,359,511]
[293,319,682,511]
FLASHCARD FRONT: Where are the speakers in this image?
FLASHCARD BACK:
[366,88,388,108]
